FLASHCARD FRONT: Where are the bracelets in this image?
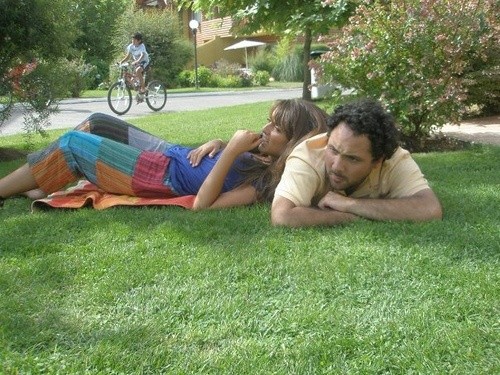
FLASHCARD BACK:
[217,138,223,151]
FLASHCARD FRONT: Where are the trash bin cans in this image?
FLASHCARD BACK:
[309,50,332,101]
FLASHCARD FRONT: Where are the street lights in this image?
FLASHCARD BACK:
[188,20,200,90]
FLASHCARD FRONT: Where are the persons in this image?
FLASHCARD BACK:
[115,32,152,104]
[0,99,332,213]
[271,102,443,226]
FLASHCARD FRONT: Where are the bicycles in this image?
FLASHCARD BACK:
[0,74,53,113]
[108,60,168,116]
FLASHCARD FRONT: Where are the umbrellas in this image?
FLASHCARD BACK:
[223,39,266,72]
